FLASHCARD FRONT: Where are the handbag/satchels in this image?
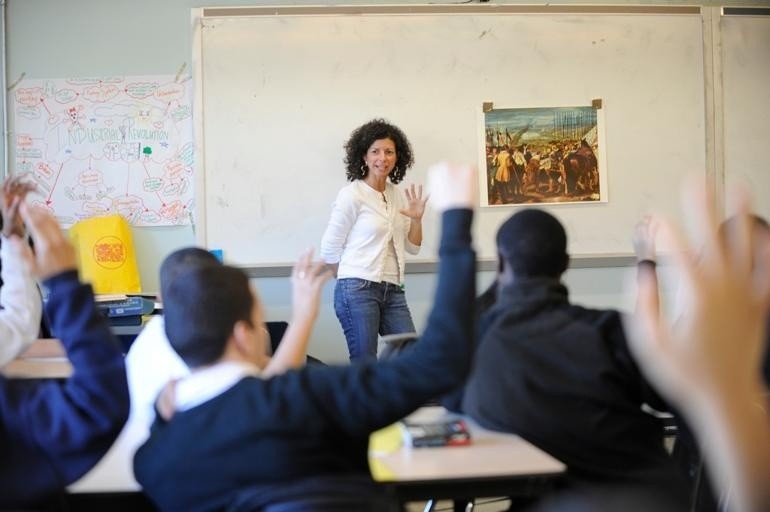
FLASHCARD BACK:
[70,216,141,296]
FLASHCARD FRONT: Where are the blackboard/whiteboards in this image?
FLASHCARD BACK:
[190,3,770,279]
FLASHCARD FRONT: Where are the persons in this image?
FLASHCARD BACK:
[0,172,481,511]
[486,142,603,205]
[441,176,770,512]
[317,120,430,364]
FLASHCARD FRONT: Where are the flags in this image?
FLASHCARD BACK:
[485,125,512,141]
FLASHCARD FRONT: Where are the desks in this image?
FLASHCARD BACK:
[367,403,569,512]
[1,337,75,379]
